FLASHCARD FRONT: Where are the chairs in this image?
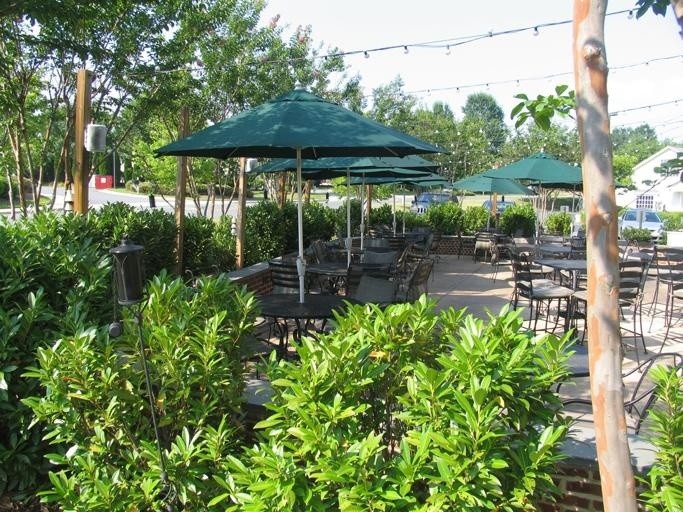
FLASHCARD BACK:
[455,224,683,435]
[240,225,441,380]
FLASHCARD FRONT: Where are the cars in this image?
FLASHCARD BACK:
[409,191,458,217]
[617,209,668,245]
[478,200,516,233]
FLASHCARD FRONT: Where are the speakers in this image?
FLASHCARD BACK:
[84,124,106,153]
